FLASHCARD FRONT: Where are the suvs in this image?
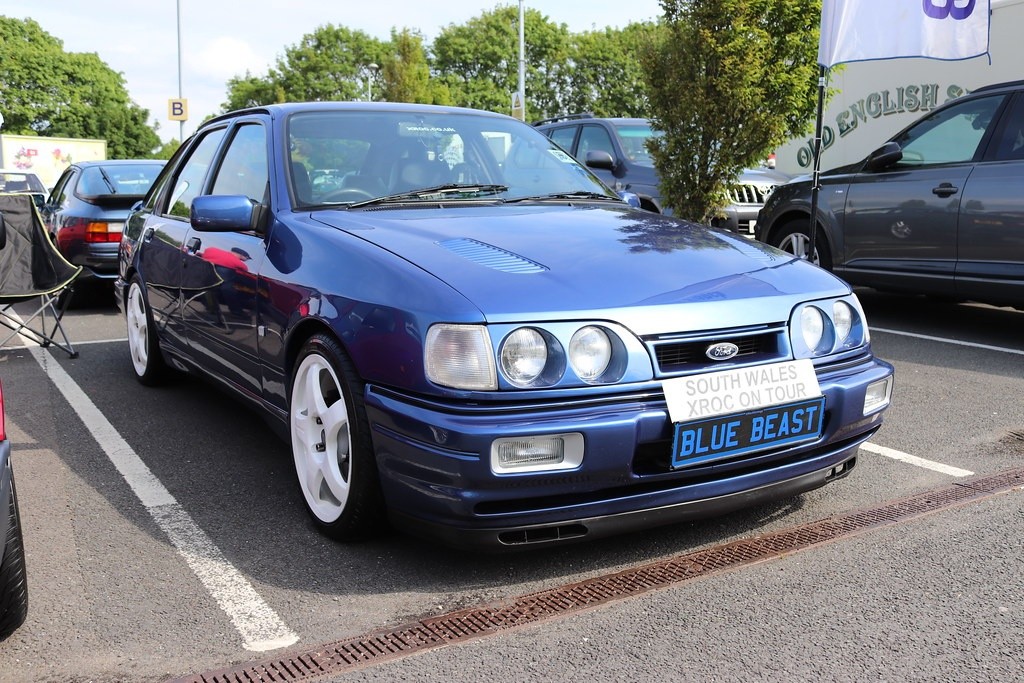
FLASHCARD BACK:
[756,80,1023,314]
[503,113,792,241]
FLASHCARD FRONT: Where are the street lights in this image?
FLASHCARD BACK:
[368,62,378,102]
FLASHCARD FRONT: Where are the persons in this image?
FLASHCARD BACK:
[766,152,776,169]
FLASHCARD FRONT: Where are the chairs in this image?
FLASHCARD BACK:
[388,142,451,196]
[291,161,313,204]
[0,194,83,358]
[136,237,233,349]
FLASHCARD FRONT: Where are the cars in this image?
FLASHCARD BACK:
[0,168,51,204]
[42,160,169,312]
[114,103,895,550]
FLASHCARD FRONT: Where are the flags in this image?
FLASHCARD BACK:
[818,0,994,70]
